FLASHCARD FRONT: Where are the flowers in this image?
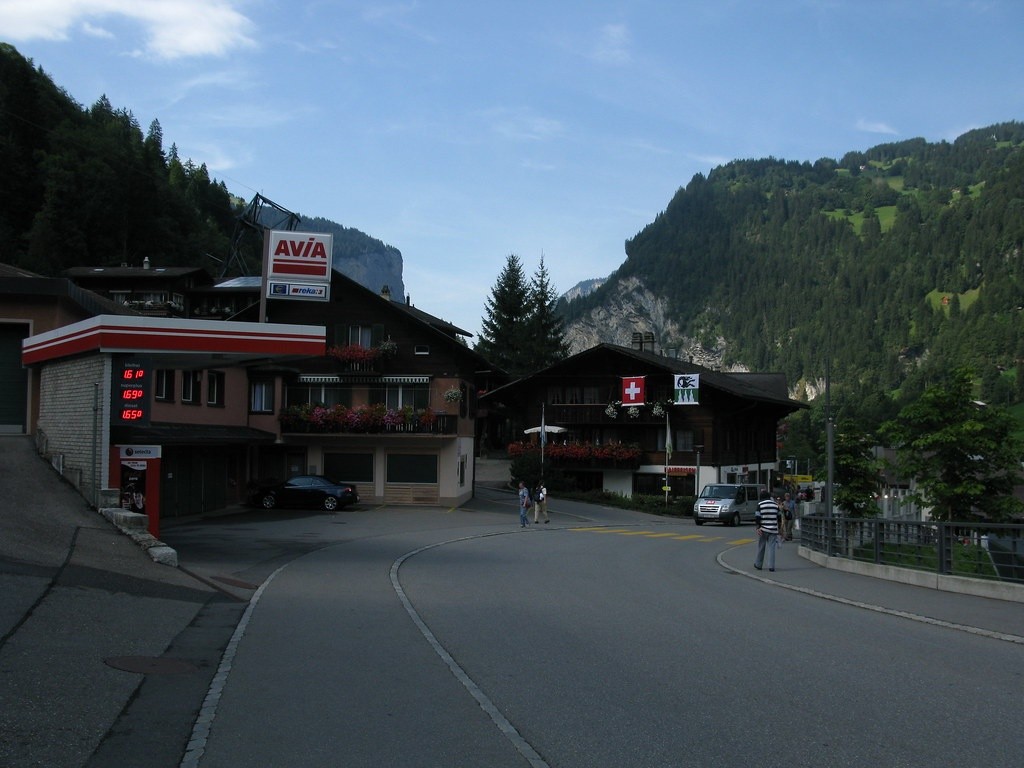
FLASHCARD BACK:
[277,400,438,430]
[507,437,646,462]
[443,385,464,403]
[378,340,400,359]
[627,406,640,418]
[327,343,378,365]
[605,401,619,419]
[652,402,665,418]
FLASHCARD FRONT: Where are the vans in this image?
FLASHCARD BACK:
[693,483,770,528]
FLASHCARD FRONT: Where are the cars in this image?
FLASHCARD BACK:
[245,473,360,512]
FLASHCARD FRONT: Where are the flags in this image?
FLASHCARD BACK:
[621,376,646,407]
[674,374,699,405]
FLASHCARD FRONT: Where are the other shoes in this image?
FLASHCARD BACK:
[769,568,774,572]
[521,524,524,527]
[527,523,530,528]
[754,563,762,570]
[535,521,539,523]
[545,520,550,524]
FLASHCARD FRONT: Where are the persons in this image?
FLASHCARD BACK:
[783,493,795,541]
[805,486,813,502]
[534,480,550,524]
[519,481,531,528]
[775,496,786,542]
[796,485,803,495]
[754,491,780,572]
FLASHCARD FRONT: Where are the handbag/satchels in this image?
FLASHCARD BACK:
[522,501,531,508]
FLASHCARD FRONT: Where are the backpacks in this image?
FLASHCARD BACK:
[534,487,544,501]
